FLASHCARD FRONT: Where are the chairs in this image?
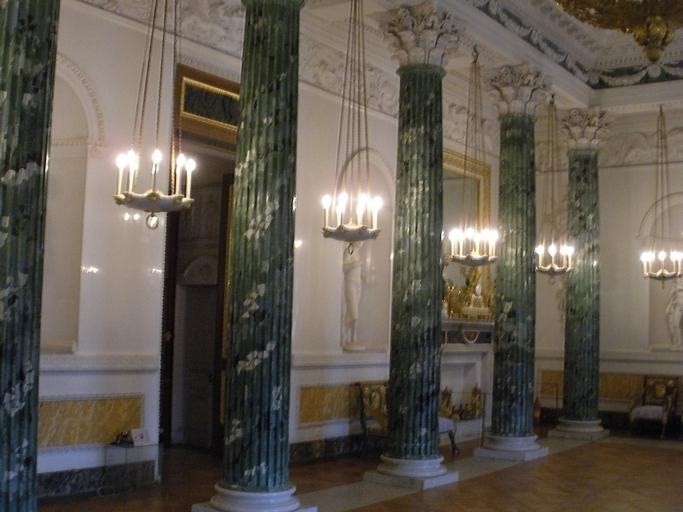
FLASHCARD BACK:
[350,382,459,458]
[627,375,680,438]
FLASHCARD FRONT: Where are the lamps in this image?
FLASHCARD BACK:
[533,94,571,277]
[320,0,383,243]
[638,103,681,279]
[109,0,197,212]
[446,48,496,267]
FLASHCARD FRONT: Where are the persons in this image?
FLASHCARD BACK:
[339,238,367,345]
